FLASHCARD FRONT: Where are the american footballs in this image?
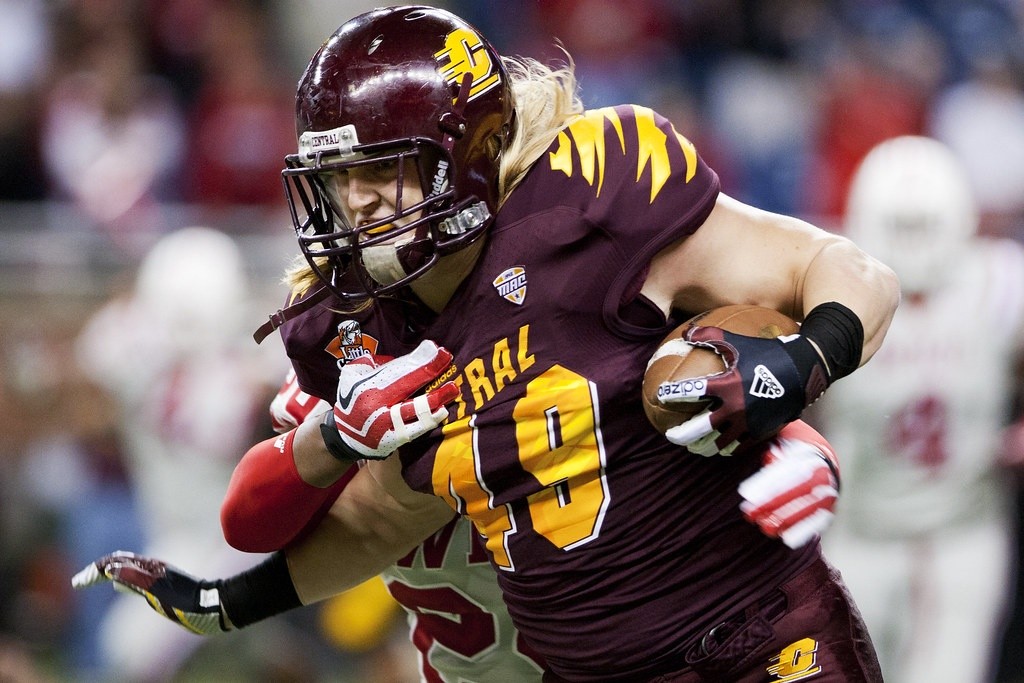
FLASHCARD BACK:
[642,304,801,434]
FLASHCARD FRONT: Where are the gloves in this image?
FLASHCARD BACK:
[320,340,459,463]
[657,324,833,461]
[734,435,838,549]
[73,550,232,636]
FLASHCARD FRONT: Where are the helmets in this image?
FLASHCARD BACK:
[281,3,515,301]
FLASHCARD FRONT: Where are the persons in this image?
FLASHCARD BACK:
[77,224,293,682]
[220,339,841,683]
[71,4,901,683]
[801,135,1024,683]
